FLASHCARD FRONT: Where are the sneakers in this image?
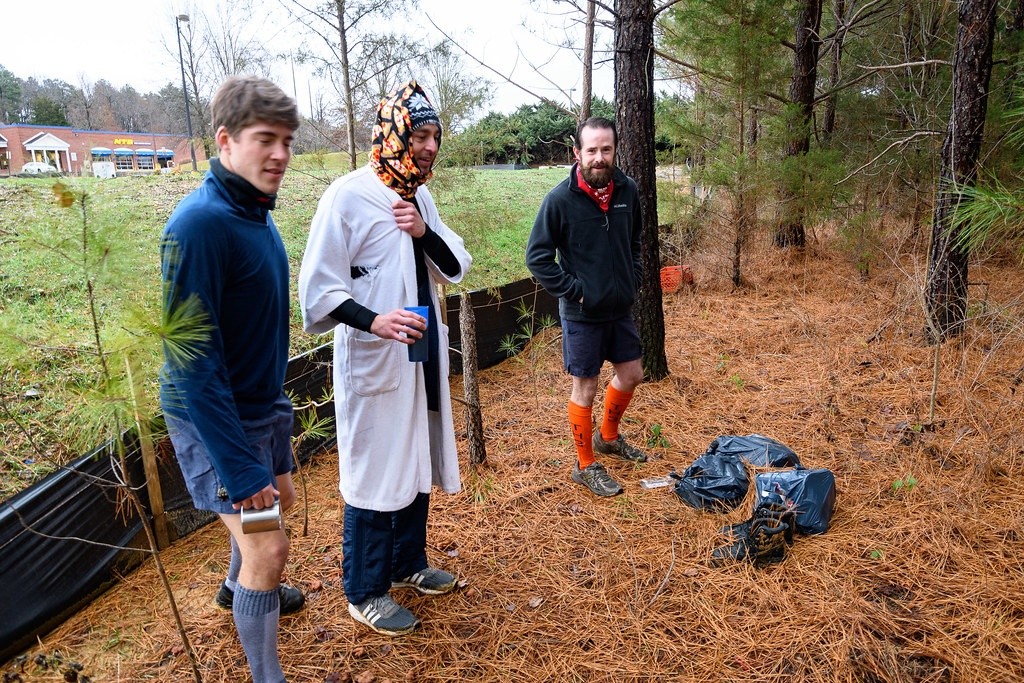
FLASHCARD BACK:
[216,579,305,614]
[720,502,794,547]
[391,567,458,595]
[571,459,624,497]
[711,518,789,563]
[592,430,647,462]
[348,593,422,636]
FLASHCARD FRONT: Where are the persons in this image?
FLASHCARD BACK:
[158,78,305,683]
[297,79,474,636]
[526,114,647,497]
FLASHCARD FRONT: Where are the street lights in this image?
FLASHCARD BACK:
[176,14,198,171]
[569,88,576,111]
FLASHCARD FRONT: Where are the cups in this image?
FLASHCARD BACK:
[241,496,282,534]
[404,306,429,362]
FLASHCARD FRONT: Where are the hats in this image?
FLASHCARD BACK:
[407,92,444,131]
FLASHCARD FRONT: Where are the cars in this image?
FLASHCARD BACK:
[22,162,56,174]
